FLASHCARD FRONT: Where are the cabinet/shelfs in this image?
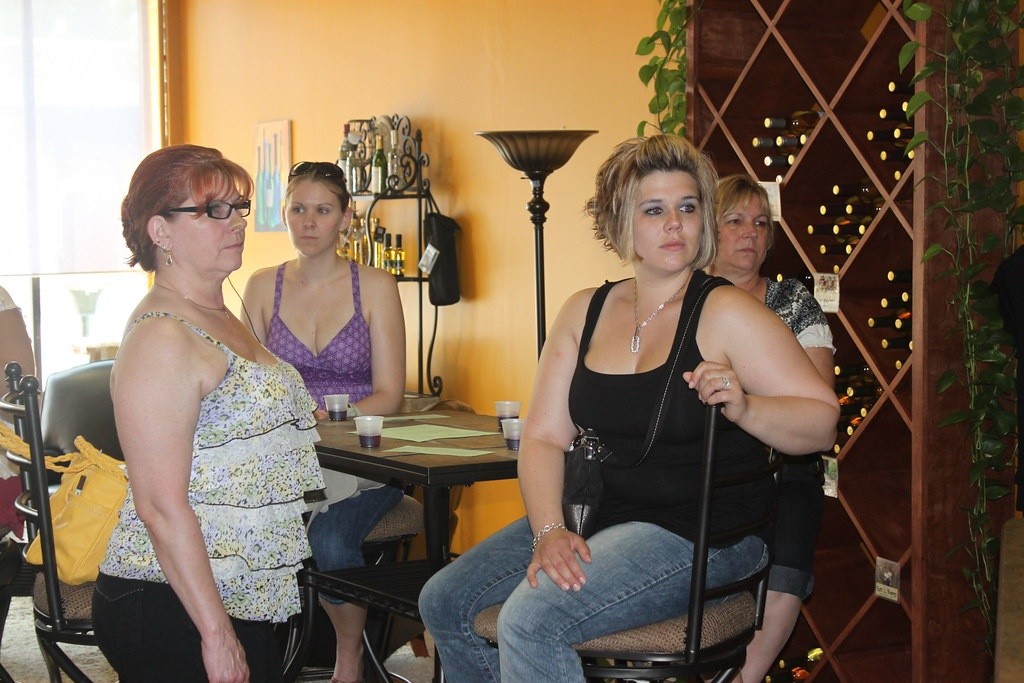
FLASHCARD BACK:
[334,112,461,398]
[686,0,1018,683]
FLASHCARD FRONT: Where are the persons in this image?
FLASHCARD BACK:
[879,567,892,586]
[701,172,836,683]
[91,145,327,683]
[0,286,36,646]
[240,161,405,683]
[418,134,840,683]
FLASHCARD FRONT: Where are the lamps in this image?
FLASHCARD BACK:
[477,129,599,362]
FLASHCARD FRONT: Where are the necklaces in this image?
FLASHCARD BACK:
[630,275,686,354]
[154,282,230,319]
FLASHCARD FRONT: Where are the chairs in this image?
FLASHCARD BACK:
[473,403,778,683]
[285,393,476,670]
[0,361,124,683]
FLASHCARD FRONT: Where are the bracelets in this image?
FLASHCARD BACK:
[531,523,567,552]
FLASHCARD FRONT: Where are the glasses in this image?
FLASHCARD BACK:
[287,161,345,186]
[169,198,251,219]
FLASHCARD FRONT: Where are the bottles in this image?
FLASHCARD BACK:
[747,77,922,461]
[388,128,403,195]
[335,201,405,277]
[757,648,824,683]
[372,135,388,195]
[337,125,353,192]
[346,130,362,194]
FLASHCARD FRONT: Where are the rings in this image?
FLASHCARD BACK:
[721,377,730,391]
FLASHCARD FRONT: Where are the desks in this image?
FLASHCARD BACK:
[296,393,520,683]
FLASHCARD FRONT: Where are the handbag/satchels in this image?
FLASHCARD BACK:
[0,422,128,586]
[560,427,605,538]
[419,190,461,307]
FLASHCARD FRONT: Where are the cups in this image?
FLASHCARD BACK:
[495,401,521,432]
[323,395,349,421]
[353,416,384,448]
[499,418,523,450]
